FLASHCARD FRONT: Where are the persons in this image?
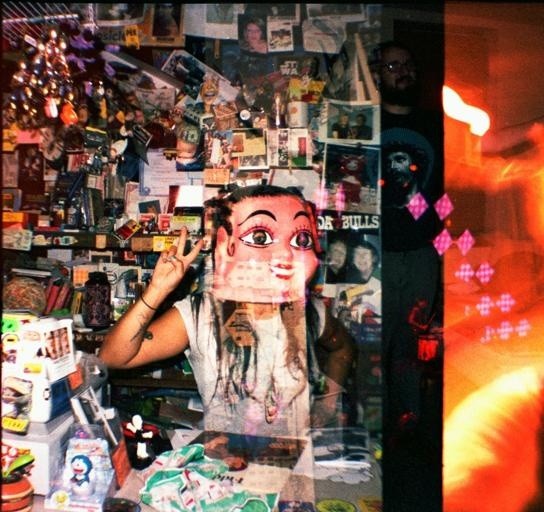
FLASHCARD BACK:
[0,0,443,511]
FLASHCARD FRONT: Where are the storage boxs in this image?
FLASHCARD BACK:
[1,417,76,497]
